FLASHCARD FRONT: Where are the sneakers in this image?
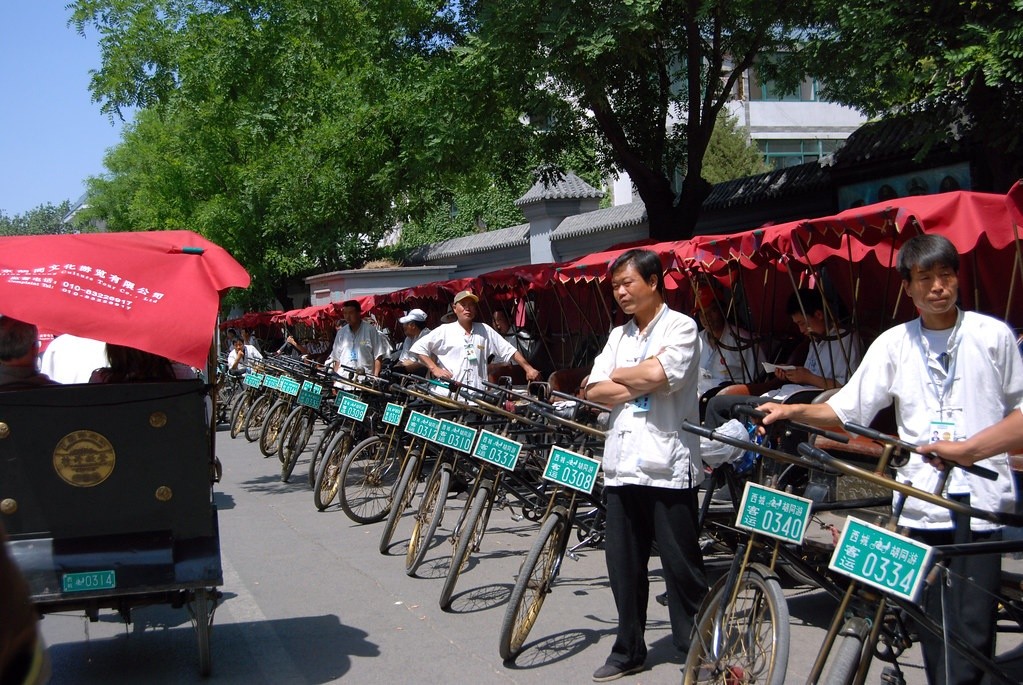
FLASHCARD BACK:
[592,662,645,682]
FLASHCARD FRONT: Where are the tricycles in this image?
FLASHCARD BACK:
[1,229,223,685]
[215,180,1022,685]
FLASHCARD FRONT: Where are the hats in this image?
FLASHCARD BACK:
[454,290,479,303]
[399,308,428,323]
[691,287,723,315]
[440,303,457,322]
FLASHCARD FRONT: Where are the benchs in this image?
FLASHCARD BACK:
[811,430,1023,472]
[548,368,605,412]
[487,362,539,395]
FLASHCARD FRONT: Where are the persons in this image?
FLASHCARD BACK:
[584,249,710,682]
[699,289,868,518]
[408,290,541,500]
[0,314,196,390]
[749,234,1023,685]
[264,319,350,364]
[327,309,434,378]
[224,329,263,380]
[691,287,769,424]
[915,401,1023,471]
[487,312,536,385]
[932,431,940,441]
[324,300,386,461]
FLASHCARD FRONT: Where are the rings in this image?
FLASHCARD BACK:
[788,375,792,379]
